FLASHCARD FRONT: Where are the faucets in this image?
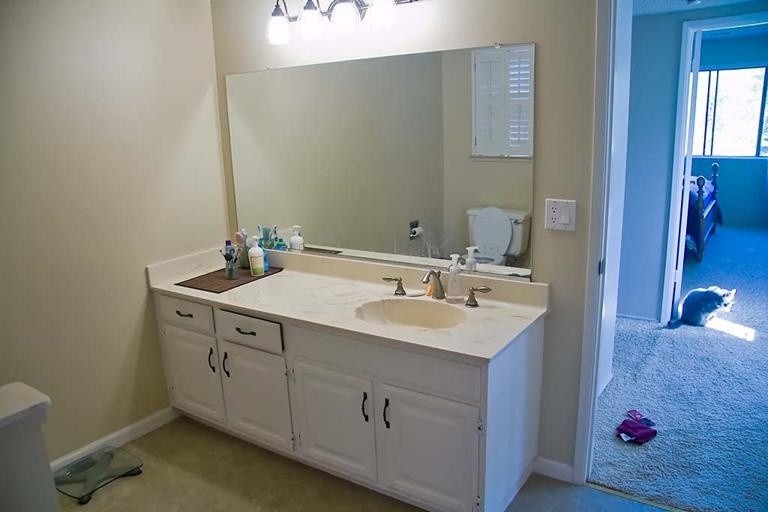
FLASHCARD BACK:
[421,269,444,300]
[449,257,466,268]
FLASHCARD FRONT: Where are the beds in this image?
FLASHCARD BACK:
[684,162,725,261]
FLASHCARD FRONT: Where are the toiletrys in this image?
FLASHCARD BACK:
[466,246,480,270]
[444,254,464,303]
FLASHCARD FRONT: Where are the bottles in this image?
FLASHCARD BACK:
[240,229,247,246]
[223,240,234,261]
[261,227,288,251]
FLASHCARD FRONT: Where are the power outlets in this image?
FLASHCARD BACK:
[544,197,578,235]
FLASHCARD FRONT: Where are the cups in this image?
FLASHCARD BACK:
[237,246,250,269]
[223,263,240,280]
[262,250,269,272]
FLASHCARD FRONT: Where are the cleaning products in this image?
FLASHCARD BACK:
[258,238,271,270]
[224,240,236,255]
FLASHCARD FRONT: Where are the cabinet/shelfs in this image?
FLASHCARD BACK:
[154,290,290,459]
[288,318,544,511]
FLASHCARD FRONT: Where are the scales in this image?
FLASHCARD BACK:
[52,445,144,505]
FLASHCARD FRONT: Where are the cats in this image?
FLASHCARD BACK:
[667,286,737,328]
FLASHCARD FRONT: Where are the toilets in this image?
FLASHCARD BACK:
[463,205,531,265]
[0,381,61,512]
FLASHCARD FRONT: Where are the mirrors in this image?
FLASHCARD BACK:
[222,40,541,279]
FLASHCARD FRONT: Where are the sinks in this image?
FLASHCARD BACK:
[354,295,468,331]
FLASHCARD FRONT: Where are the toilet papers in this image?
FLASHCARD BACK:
[412,227,423,237]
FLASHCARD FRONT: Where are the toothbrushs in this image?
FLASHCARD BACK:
[257,223,279,238]
[219,249,241,263]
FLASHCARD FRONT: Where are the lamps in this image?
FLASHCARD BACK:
[264,1,417,38]
